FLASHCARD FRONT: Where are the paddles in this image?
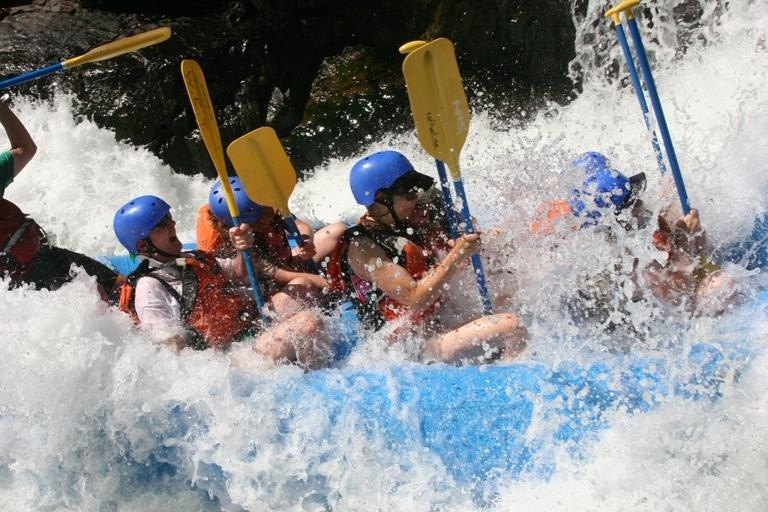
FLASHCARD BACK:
[179,60,269,331]
[226,127,320,275]
[0,25,170,87]
[403,36,491,316]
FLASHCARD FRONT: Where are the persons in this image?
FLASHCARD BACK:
[196,176,348,298]
[328,150,528,363]
[564,171,738,354]
[0,96,117,304]
[531,152,610,236]
[112,194,328,372]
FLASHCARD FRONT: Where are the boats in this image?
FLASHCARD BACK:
[69,244,768,498]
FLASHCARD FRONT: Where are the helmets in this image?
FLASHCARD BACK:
[349,151,435,207]
[208,175,261,227]
[568,169,647,231]
[558,153,608,192]
[113,196,172,255]
